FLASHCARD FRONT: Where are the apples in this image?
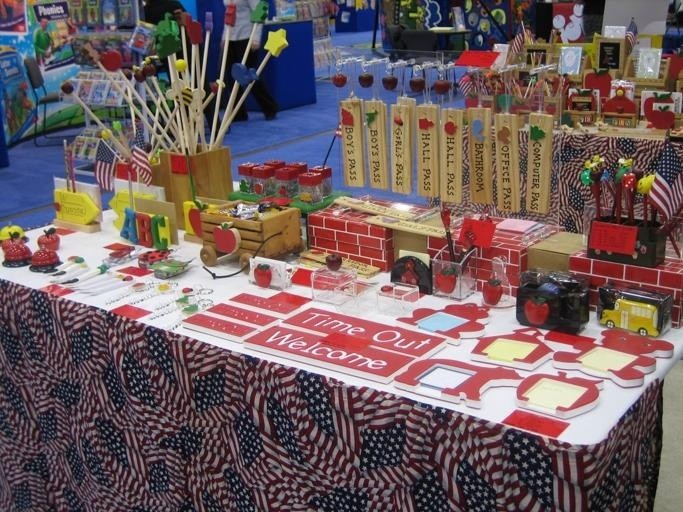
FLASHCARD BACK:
[326,254,343,270]
[37,228,59,251]
[4,242,31,261]
[466,51,683,129]
[214,222,242,254]
[0,223,24,240]
[434,266,457,294]
[332,73,450,93]
[483,279,503,305]
[254,263,272,287]
[524,296,549,325]
[189,199,208,237]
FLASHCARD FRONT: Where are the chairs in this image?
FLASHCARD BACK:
[23,56,80,147]
[387,23,457,98]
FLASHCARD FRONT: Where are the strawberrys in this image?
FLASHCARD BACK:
[32,249,59,267]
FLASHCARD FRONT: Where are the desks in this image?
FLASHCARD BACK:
[0,182,683,511]
[428,26,472,71]
[425,116,683,257]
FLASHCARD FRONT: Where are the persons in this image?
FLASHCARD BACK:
[142,0,222,138]
[224,0,277,122]
[562,48,576,73]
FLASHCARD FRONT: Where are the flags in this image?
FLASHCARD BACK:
[626,20,639,48]
[647,140,683,222]
[476,74,491,95]
[510,24,524,56]
[459,75,474,97]
[93,139,117,194]
[130,128,152,188]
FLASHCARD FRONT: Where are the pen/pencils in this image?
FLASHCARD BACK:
[63,139,75,192]
